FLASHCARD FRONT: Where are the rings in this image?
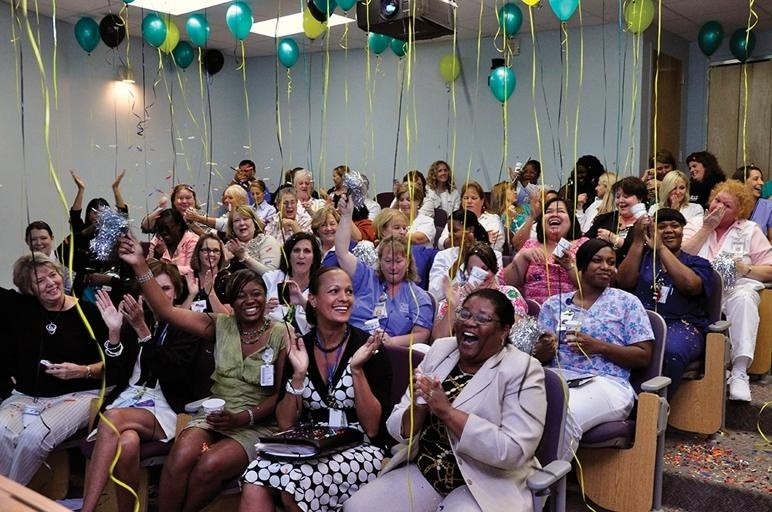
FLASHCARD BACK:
[578,342,582,347]
[372,349,380,354]
[429,390,434,397]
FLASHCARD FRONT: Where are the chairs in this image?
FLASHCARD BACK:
[747,283,772,380]
[25,429,88,502]
[526,367,571,512]
[576,310,672,512]
[77,435,175,512]
[363,345,425,458]
[669,269,731,440]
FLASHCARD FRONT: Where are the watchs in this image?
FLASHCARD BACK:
[285,379,305,396]
[85,366,92,379]
[247,409,255,428]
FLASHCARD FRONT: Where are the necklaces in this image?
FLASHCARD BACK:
[314,327,349,352]
[239,318,270,335]
[239,318,271,344]
[317,331,349,397]
[45,296,65,336]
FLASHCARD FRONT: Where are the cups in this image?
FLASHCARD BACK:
[23,406,41,427]
[565,321,582,332]
[552,237,572,259]
[629,203,647,219]
[364,318,381,337]
[467,266,489,289]
[202,398,226,419]
[514,162,522,173]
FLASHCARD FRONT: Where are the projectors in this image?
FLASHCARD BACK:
[357,2,457,43]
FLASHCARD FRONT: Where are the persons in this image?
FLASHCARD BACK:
[544,190,559,206]
[0,252,122,485]
[225,203,281,275]
[431,240,529,341]
[335,170,433,345]
[180,184,249,234]
[501,198,591,314]
[537,237,654,462]
[327,165,352,206]
[76,258,213,512]
[249,179,277,227]
[680,177,772,401]
[686,151,728,207]
[586,176,651,264]
[231,159,271,205]
[572,173,620,235]
[429,209,503,296]
[373,207,439,290]
[118,227,287,512]
[639,148,676,207]
[505,159,553,206]
[259,232,319,308]
[343,289,547,512]
[559,154,606,220]
[423,159,461,213]
[261,186,314,239]
[649,169,704,248]
[145,208,201,310]
[615,209,713,403]
[729,167,771,245]
[489,182,533,236]
[23,221,56,258]
[387,171,434,213]
[311,207,359,273]
[238,266,395,512]
[394,181,436,246]
[178,234,233,316]
[459,181,505,249]
[64,167,129,257]
[359,173,382,220]
[290,170,330,216]
[140,185,202,255]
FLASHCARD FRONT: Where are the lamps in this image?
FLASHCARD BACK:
[118,67,135,84]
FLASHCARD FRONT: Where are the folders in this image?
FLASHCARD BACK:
[259,423,364,460]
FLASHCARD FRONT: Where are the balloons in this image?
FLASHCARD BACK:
[336,0,355,12]
[76,17,102,56]
[440,53,462,85]
[521,0,541,6]
[698,20,724,60]
[141,12,167,48]
[498,4,523,39]
[226,1,255,41]
[368,29,388,56]
[204,49,224,76]
[122,0,135,4]
[276,38,300,68]
[185,13,211,47]
[159,19,180,56]
[624,0,654,36]
[548,0,579,24]
[489,66,516,106]
[173,40,194,72]
[307,0,330,23]
[389,35,413,58]
[729,26,756,64]
[303,7,325,39]
[315,0,336,17]
[99,12,127,48]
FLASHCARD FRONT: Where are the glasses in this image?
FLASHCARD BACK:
[455,309,508,326]
[200,248,221,254]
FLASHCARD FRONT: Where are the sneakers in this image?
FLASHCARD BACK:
[730,369,752,402]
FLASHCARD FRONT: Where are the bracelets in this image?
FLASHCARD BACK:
[743,264,751,277]
[137,269,153,283]
[104,339,123,357]
